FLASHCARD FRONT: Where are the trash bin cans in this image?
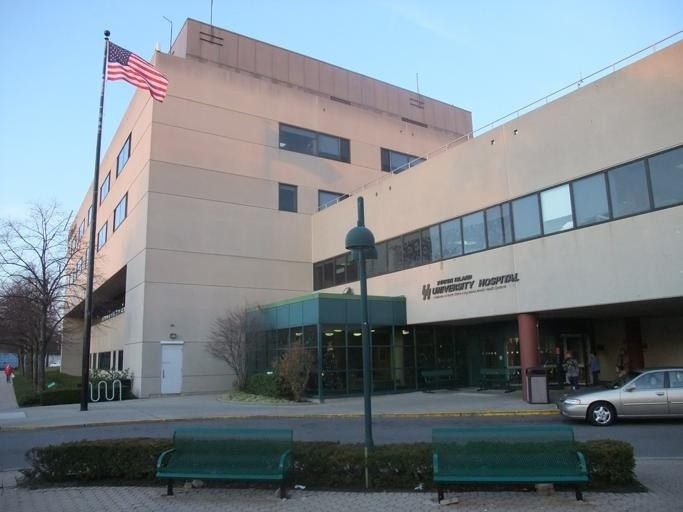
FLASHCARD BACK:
[526,367,550,404]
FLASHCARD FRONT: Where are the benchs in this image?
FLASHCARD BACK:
[156,426,297,499]
[432,427,589,504]
[421,369,461,393]
[476,368,516,393]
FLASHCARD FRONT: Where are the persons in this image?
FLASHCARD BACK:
[615,348,630,378]
[566,352,580,389]
[590,352,600,386]
[4,364,15,383]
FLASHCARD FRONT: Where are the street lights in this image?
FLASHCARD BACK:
[344,195,379,489]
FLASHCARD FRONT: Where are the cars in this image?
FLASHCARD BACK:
[556,366,682,427]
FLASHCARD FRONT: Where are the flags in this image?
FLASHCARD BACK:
[106,40,169,103]
